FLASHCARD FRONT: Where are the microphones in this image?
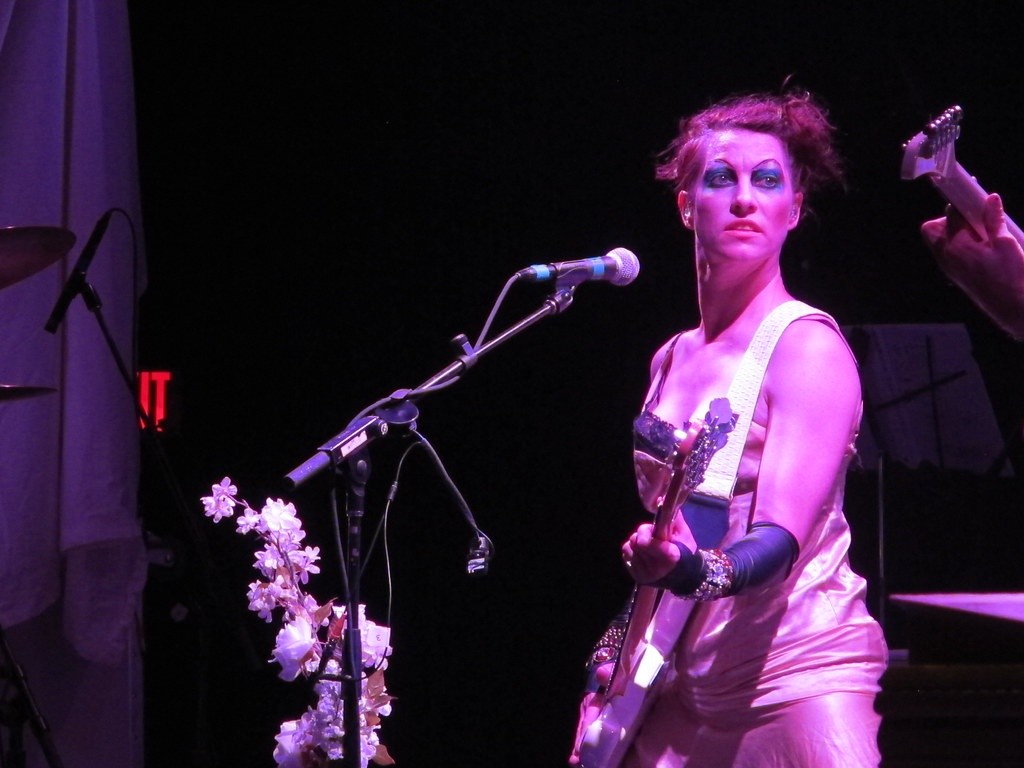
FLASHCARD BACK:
[43,211,112,335]
[518,247,640,287]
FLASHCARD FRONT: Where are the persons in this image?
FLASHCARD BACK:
[570,99,889,768]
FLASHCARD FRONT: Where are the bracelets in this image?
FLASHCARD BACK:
[588,627,625,673]
[689,547,733,601]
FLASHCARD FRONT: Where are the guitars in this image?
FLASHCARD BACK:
[577,418,704,768]
[900,101,1024,246]
